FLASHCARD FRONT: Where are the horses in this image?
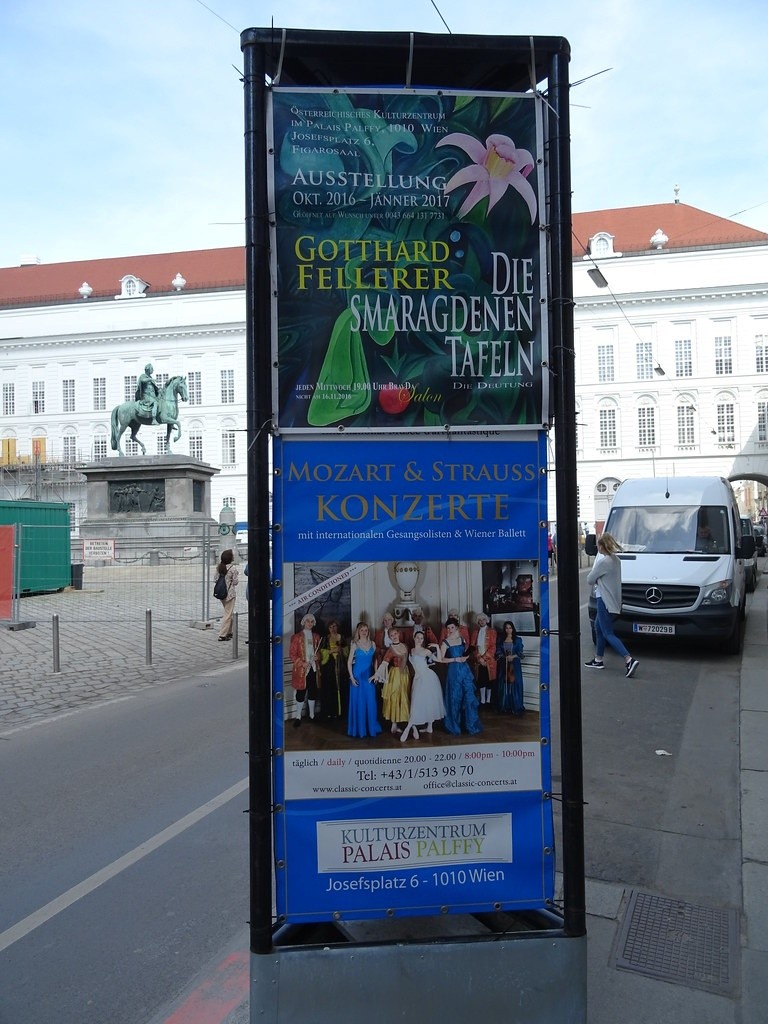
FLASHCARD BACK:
[110,375,188,458]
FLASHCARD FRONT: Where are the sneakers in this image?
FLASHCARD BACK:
[584,658,605,669]
[625,658,639,677]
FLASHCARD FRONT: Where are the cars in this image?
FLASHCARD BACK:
[752,524,768,556]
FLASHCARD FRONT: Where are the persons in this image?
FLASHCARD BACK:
[583,534,640,678]
[243,562,249,644]
[135,363,160,426]
[212,549,239,642]
[548,526,557,566]
[291,612,526,742]
[696,524,716,546]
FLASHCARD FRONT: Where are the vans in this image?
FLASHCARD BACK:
[740,515,763,592]
[584,475,755,657]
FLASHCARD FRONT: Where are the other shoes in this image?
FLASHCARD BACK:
[294,716,302,727]
[479,703,491,708]
[309,715,320,722]
[400,731,409,742]
[413,731,419,740]
[391,728,402,733]
[218,633,233,641]
[419,729,433,733]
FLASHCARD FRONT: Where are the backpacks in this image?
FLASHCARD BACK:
[213,565,233,599]
